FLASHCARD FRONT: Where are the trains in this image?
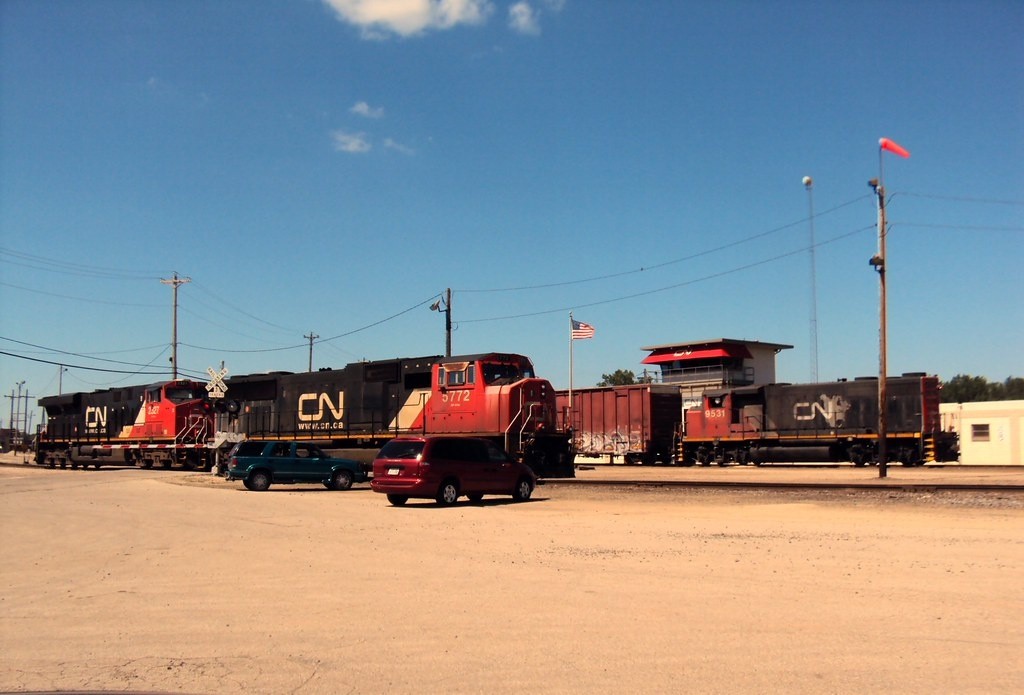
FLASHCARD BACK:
[32,350,576,478]
[553,370,959,465]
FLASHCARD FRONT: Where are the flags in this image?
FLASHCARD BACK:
[572,319,594,340]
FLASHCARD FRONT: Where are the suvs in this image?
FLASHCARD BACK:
[371,436,535,509]
[227,442,370,492]
